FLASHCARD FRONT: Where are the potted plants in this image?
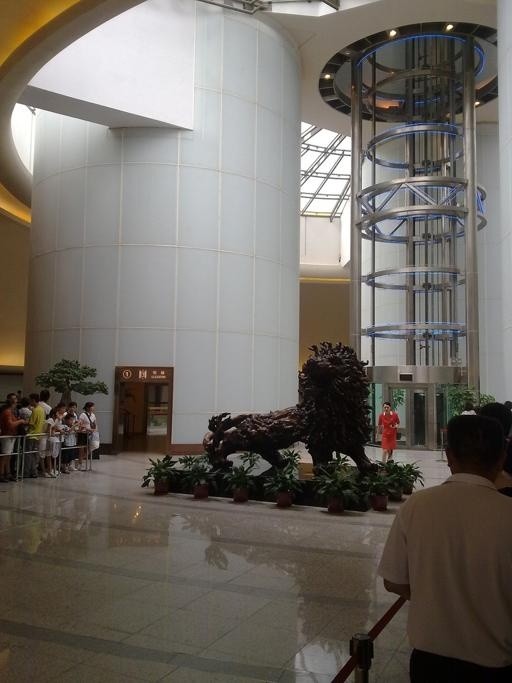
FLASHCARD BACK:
[225,449,261,502]
[139,453,182,494]
[313,454,355,512]
[262,450,305,506]
[179,454,222,498]
[355,460,425,511]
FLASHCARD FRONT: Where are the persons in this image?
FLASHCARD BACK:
[0,390,100,479]
[375,415,512,683]
[454,399,512,498]
[375,402,401,465]
